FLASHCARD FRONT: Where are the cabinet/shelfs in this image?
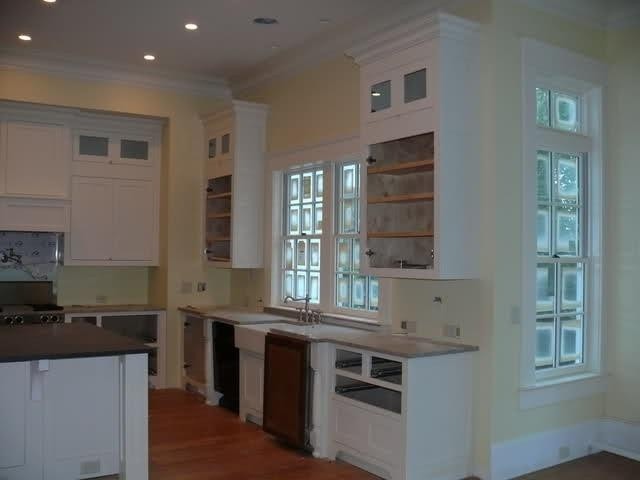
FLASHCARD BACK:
[177,304,283,413]
[63,118,162,267]
[198,97,270,269]
[231,320,481,480]
[64,305,166,391]
[357,36,473,280]
[0,119,64,234]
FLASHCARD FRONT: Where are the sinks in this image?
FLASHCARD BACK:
[233,323,308,355]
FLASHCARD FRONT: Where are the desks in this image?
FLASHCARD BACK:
[0,321,157,480]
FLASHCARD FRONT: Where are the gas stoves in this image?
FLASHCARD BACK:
[2,302,65,315]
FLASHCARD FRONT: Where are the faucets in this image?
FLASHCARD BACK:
[283,294,312,323]
